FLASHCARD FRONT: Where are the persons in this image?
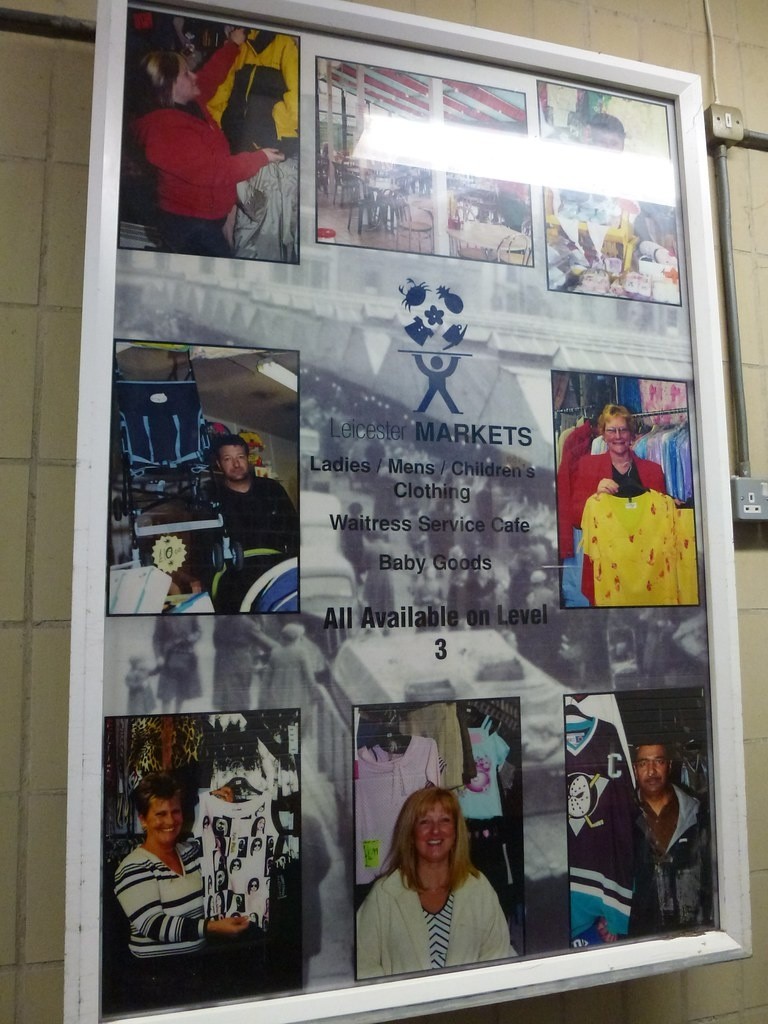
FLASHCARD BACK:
[573,404,686,607]
[192,435,300,614]
[356,786,520,979]
[132,26,301,257]
[124,413,559,747]
[544,112,679,276]
[114,772,250,1006]
[629,734,716,940]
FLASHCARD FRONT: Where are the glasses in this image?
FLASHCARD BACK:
[602,427,629,433]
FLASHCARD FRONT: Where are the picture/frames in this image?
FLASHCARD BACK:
[63,1,754,1024]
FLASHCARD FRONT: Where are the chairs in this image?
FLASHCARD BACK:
[318,142,434,253]
[454,205,532,265]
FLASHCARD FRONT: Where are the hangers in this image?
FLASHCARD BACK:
[190,759,290,816]
[631,407,689,433]
[468,701,515,737]
[565,695,593,720]
[368,706,410,754]
[618,461,649,494]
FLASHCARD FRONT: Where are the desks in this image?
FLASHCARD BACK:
[454,221,526,252]
